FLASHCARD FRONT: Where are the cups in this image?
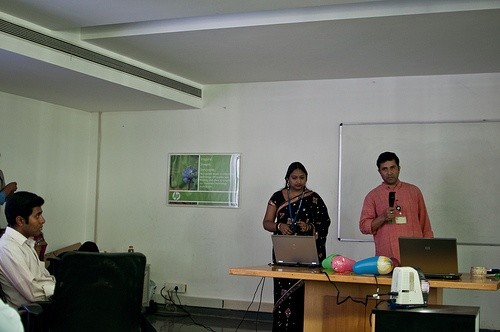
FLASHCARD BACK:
[471,266,487,276]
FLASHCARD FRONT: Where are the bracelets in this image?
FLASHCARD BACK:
[277,222,281,229]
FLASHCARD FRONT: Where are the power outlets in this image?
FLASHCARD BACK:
[171,283,186,293]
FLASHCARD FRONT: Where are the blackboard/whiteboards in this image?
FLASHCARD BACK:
[336,119,500,246]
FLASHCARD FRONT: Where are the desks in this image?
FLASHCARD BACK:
[229,265,500,332]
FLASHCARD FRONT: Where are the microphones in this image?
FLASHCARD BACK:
[388,192,396,211]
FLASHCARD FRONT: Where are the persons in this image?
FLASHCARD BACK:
[0,165,157,331]
[263,161,331,332]
[358,151,435,266]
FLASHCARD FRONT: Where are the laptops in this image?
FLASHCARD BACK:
[399,237,463,280]
[271,234,323,267]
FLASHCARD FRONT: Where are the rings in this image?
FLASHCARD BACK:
[390,211,393,213]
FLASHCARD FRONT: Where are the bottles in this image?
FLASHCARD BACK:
[128,246,134,253]
